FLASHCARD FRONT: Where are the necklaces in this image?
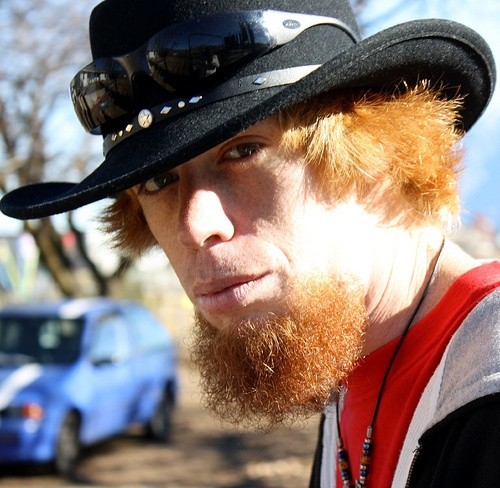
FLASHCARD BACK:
[335,237,447,488]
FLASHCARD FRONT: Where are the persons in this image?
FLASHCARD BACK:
[1,0,500,488]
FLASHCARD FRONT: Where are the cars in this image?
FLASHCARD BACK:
[0,300,176,482]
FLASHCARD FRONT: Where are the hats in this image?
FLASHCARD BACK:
[0,0,495,221]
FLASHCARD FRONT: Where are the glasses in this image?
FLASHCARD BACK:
[66,10,375,137]
